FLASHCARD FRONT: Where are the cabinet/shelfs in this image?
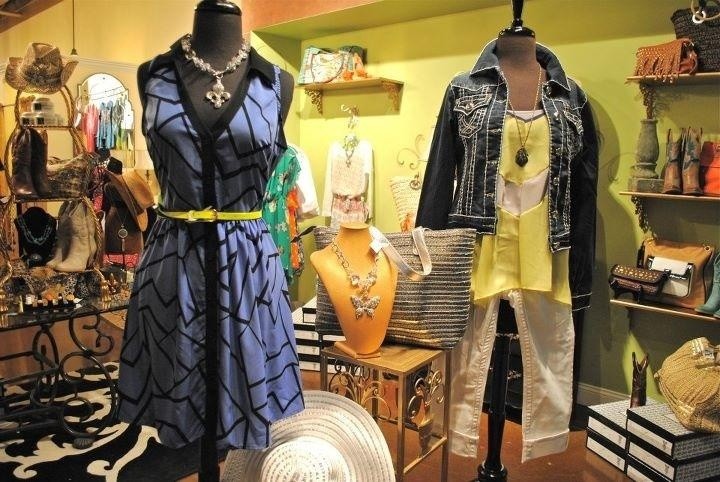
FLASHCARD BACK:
[0,81,139,450]
[610,70,720,323]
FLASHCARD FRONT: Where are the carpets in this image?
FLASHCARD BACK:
[0,358,228,481]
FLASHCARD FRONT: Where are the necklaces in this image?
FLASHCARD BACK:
[17,212,55,247]
[497,56,543,165]
[179,30,253,105]
[343,134,357,168]
[329,240,388,323]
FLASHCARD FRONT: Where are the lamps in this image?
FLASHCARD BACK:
[68,0,80,60]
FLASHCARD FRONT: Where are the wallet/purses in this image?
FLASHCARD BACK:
[610,264,671,296]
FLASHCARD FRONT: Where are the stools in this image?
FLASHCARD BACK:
[321,341,453,482]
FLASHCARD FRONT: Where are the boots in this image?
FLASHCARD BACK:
[12,124,53,200]
[661,126,704,196]
[695,253,720,319]
[45,198,97,272]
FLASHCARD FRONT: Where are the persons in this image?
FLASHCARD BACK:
[416,0,600,461]
[115,0,306,453]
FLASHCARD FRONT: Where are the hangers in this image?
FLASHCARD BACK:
[335,117,360,144]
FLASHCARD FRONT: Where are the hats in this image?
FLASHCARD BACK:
[104,168,155,232]
[220,390,396,482]
[5,42,79,94]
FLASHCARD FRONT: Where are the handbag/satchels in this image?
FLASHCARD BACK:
[388,174,457,232]
[700,141,720,197]
[46,151,102,199]
[654,337,720,433]
[637,237,716,310]
[297,45,365,85]
[635,0,720,83]
[315,225,477,351]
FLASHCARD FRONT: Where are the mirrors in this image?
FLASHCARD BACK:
[71,72,137,169]
